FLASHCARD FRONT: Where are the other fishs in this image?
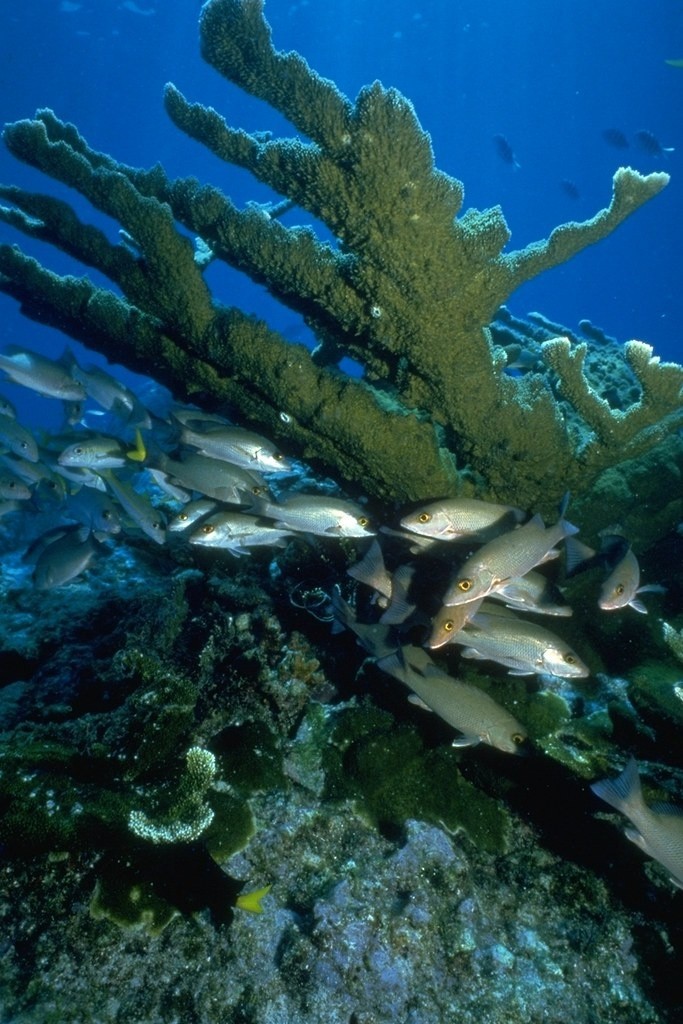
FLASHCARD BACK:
[1,335,682,893]
[493,126,674,206]
